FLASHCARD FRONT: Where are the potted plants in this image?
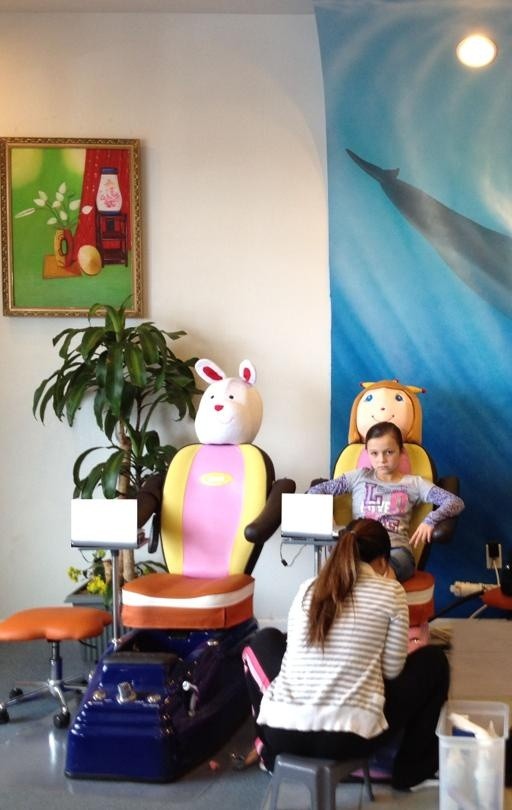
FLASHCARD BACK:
[33,294,206,683]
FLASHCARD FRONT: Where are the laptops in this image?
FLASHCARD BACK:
[281,493,346,540]
[70,498,149,548]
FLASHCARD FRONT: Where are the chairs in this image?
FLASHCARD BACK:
[119,443,297,631]
[301,443,460,634]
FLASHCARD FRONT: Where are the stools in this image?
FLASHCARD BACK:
[268,755,375,810]
[0,606,112,727]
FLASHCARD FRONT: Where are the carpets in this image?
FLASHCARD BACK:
[0,638,362,809]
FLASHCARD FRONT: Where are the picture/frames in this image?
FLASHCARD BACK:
[3,137,147,318]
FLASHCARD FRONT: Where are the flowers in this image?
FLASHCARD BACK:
[64,549,116,600]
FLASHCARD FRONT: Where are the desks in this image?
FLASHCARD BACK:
[280,537,341,578]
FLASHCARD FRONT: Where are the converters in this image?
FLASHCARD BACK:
[488,543,499,558]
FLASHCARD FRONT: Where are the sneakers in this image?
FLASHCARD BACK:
[393,765,439,791]
[258,754,275,776]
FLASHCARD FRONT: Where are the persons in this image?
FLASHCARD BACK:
[306,421,467,585]
[242,516,451,792]
